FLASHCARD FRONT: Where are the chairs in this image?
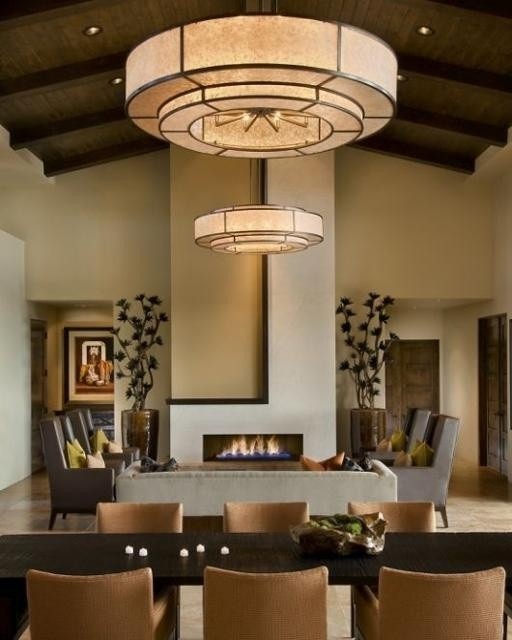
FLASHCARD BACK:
[40,416,126,530]
[97,502,181,640]
[381,414,459,527]
[67,408,140,467]
[223,501,310,533]
[348,501,435,638]
[26,568,177,640]
[356,565,507,640]
[204,566,329,640]
[362,407,432,459]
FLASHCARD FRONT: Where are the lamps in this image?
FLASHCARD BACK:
[125,0,398,159]
[194,159,324,255]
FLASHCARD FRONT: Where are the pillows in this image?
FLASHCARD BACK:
[376,437,392,452]
[394,450,412,467]
[86,451,106,469]
[300,451,373,472]
[89,427,110,454]
[391,425,406,452]
[411,438,434,466]
[67,438,86,468]
[107,440,123,454]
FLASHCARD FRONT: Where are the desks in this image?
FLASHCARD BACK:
[0,532,512,640]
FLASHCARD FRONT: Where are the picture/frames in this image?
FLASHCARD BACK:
[63,326,115,411]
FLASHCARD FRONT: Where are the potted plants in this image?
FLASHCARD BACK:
[110,293,170,465]
[335,292,400,459]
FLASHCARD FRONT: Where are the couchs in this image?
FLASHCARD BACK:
[116,459,398,517]
